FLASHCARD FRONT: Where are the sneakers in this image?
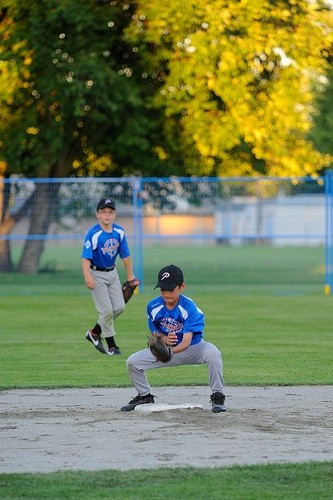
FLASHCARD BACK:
[209,392,226,413]
[121,394,155,411]
[85,330,107,353]
[106,346,122,355]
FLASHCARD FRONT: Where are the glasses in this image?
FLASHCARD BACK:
[98,210,114,216]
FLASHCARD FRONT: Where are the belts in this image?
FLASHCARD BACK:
[90,265,114,272]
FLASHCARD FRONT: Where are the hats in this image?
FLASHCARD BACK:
[154,264,184,290]
[97,197,115,210]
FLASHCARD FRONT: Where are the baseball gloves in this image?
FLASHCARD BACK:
[121,277,142,305]
[144,329,174,363]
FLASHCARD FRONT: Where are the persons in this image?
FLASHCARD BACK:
[120,264,226,413]
[81,198,140,356]
